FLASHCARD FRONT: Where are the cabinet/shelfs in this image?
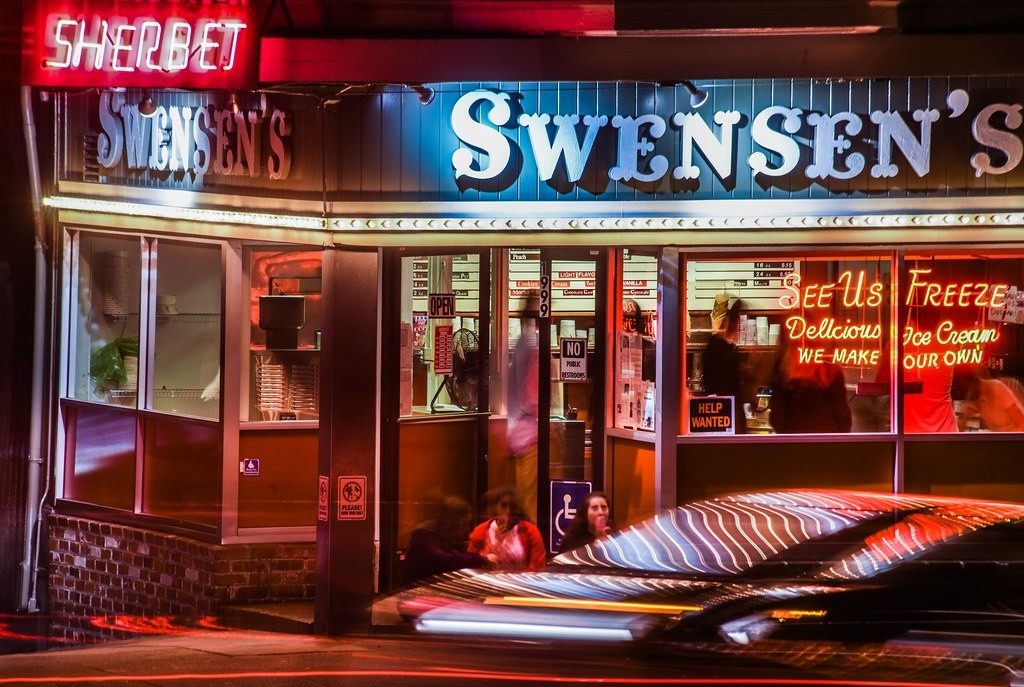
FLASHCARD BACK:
[413,312,797,458]
[99,312,221,399]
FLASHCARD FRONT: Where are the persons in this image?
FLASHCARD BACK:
[558,492,616,556]
[769,313,853,434]
[623,298,656,383]
[506,290,553,524]
[875,293,1024,433]
[398,498,502,585]
[698,295,758,434]
[468,488,546,570]
